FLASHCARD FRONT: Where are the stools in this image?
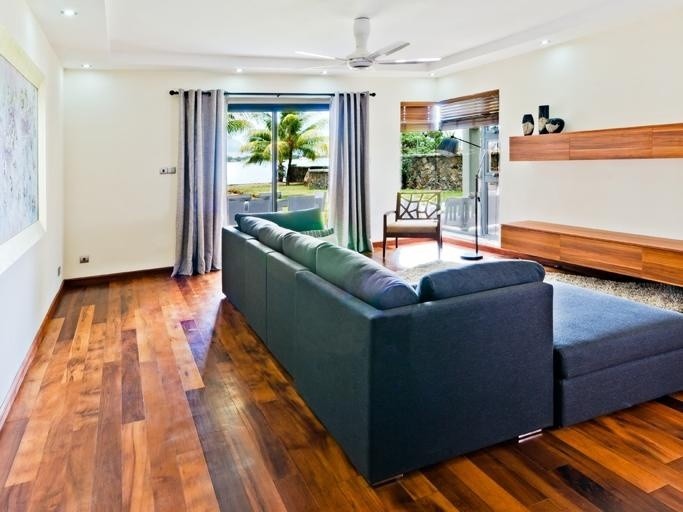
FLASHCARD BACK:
[542,278,683,428]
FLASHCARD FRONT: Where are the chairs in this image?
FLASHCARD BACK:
[383,191,442,261]
[228,191,326,225]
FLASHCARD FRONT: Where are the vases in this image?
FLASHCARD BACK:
[545,118,564,133]
[521,114,534,136]
[538,106,550,134]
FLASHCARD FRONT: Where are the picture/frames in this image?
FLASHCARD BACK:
[0,32,47,276]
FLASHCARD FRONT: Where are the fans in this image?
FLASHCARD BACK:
[296,18,441,69]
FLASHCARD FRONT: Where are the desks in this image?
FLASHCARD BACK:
[501,122,683,288]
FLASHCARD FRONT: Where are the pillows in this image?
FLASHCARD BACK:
[298,228,338,245]
[239,214,418,310]
[418,261,547,302]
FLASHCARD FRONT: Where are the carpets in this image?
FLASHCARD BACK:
[397,261,683,315]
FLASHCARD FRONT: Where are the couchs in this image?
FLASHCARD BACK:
[222,206,553,486]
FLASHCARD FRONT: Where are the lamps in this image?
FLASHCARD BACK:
[436,131,488,261]
